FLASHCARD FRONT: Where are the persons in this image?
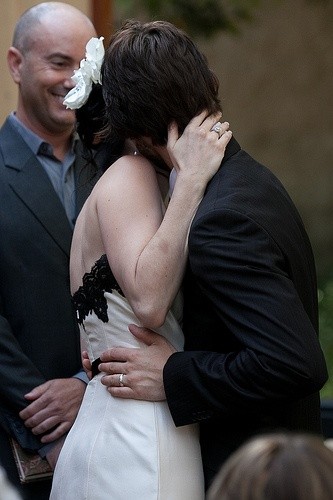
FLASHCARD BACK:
[50,36,231,500]
[0,3,98,500]
[206,433,333,500]
[82,19,329,495]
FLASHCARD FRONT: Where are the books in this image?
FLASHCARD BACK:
[11,437,54,482]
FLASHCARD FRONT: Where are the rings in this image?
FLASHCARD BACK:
[118,374,124,387]
[211,127,221,137]
